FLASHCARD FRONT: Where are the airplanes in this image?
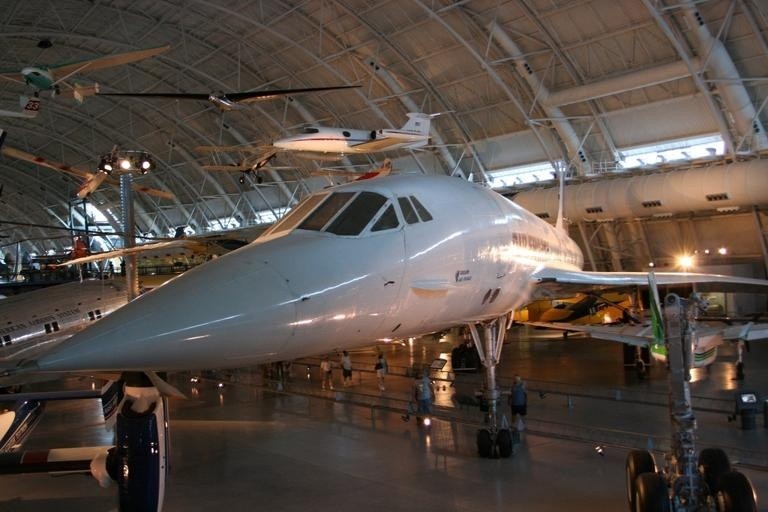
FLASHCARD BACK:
[272,111,449,157]
[0,233,250,479]
[0,158,768,512]
[1,47,172,119]
[1,142,173,206]
[92,85,364,110]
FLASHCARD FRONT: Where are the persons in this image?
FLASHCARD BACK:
[509,374,529,431]
[109,261,114,276]
[22,251,32,282]
[412,371,432,425]
[340,351,353,387]
[622,308,630,323]
[375,353,388,391]
[4,251,13,279]
[320,356,337,391]
